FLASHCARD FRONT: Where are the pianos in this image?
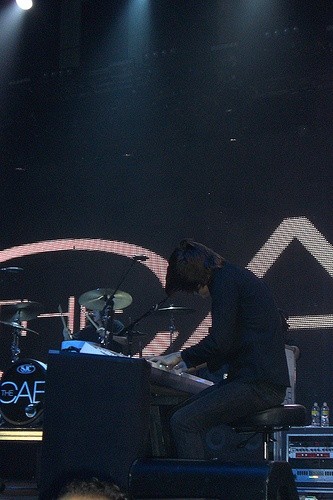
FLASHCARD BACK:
[37,339,215,500]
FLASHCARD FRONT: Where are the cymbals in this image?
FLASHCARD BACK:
[0,301,48,322]
[158,306,194,311]
[78,287,133,310]
[0,319,40,336]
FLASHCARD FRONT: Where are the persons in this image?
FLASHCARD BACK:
[57,304,130,355]
[57,472,130,500]
[150,237,292,459]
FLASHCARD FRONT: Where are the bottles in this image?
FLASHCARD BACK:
[321,402,329,427]
[311,402,320,426]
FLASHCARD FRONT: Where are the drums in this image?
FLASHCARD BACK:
[0,358,48,426]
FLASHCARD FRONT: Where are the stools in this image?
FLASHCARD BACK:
[232,404,307,461]
